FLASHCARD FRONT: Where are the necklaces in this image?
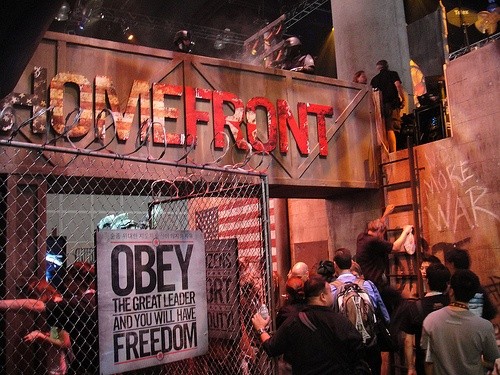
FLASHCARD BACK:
[448,302,469,311]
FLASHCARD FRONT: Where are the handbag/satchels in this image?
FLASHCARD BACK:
[347,359,375,375]
[378,317,398,352]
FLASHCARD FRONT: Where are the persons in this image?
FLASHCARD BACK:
[420,268,499,375]
[26,278,74,374]
[420,255,452,308]
[316,259,338,284]
[370,59,406,152]
[45,260,98,295]
[321,252,392,375]
[352,69,368,84]
[401,263,453,374]
[205,255,294,374]
[173,28,195,54]
[274,275,308,368]
[0,260,101,374]
[355,203,422,375]
[251,277,374,374]
[278,35,315,74]
[333,247,364,281]
[290,261,320,297]
[443,248,497,324]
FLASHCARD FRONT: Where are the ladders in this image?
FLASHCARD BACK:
[370,134,425,321]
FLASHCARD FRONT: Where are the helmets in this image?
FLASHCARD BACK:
[284,37,302,48]
[173,30,192,45]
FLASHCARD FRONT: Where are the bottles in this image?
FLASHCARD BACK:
[260,304,270,331]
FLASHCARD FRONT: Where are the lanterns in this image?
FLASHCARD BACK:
[474,9,497,35]
[447,6,477,27]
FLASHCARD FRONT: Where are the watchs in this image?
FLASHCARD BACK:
[256,327,267,335]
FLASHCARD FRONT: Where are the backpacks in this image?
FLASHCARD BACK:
[330,278,377,348]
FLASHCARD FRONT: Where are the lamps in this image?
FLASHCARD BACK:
[78,8,93,30]
[213,39,226,50]
[121,23,135,41]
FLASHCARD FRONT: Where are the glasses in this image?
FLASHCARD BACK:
[182,38,190,40]
